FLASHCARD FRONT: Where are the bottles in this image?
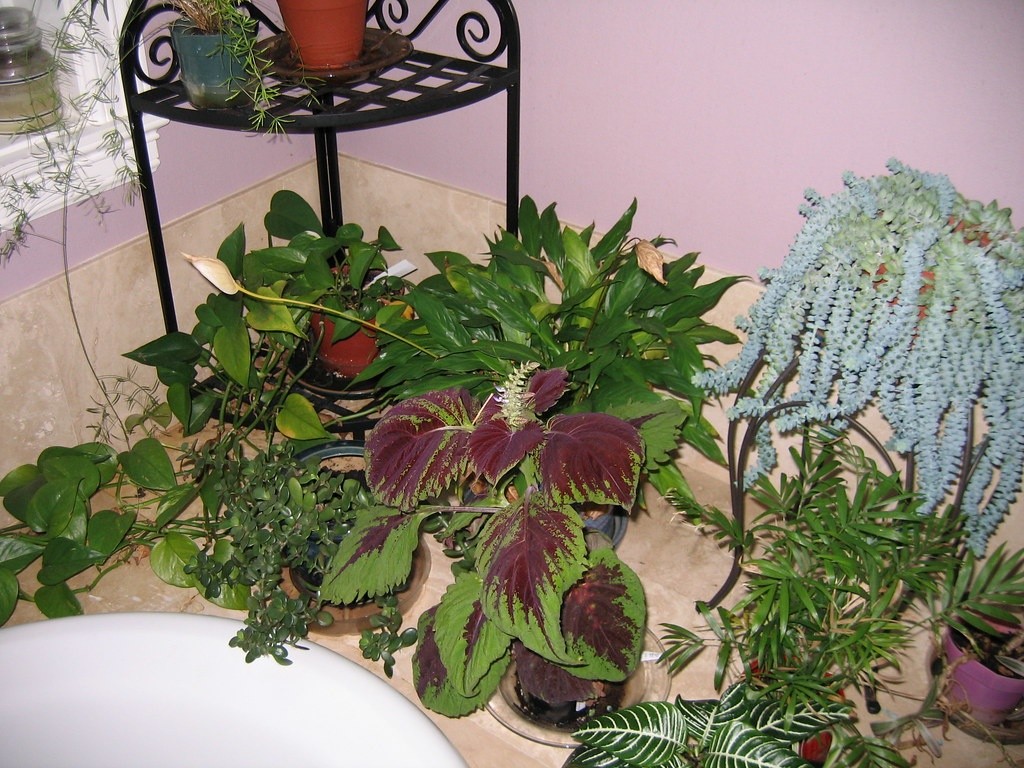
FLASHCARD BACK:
[0,7,61,134]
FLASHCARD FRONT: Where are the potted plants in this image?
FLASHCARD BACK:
[175,438,432,679]
[1,0,326,270]
[657,417,1024,767]
[926,606,1024,747]
[314,355,673,753]
[340,194,755,553]
[1,189,416,629]
[687,155,1024,564]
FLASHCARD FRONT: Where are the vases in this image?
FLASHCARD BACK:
[250,0,413,79]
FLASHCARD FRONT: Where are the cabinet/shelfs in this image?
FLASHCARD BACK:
[117,0,522,435]
